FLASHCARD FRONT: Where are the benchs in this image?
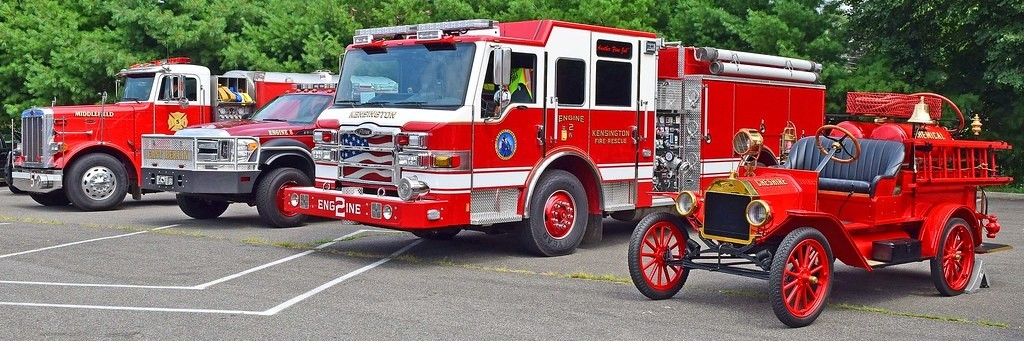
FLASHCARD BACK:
[781,135,906,192]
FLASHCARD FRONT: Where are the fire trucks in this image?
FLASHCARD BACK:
[12,57,339,212]
[284,19,826,257]
[140,88,337,228]
